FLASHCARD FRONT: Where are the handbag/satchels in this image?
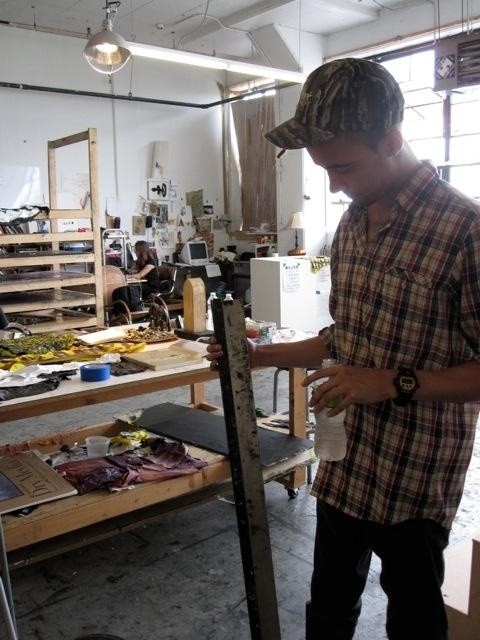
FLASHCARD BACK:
[209,251,237,265]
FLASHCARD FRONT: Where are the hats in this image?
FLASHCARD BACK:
[264,57,403,150]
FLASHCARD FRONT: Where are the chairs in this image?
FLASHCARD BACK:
[108,265,177,325]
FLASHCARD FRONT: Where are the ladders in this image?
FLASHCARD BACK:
[102,230,127,327]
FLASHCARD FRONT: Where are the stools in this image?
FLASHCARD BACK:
[273,365,323,484]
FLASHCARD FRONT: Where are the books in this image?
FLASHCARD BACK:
[121,347,203,371]
[16,249,37,253]
[0,247,8,255]
[0,225,23,236]
[0,450,79,515]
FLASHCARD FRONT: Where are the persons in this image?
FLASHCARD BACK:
[125,241,164,301]
[205,58,480,639]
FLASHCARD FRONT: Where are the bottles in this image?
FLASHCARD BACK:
[311,359,347,462]
[207,292,217,331]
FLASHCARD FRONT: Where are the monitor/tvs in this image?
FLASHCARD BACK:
[181,241,208,264]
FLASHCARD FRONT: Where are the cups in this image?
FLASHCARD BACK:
[85,436,110,457]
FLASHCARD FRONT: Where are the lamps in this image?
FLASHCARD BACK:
[126,0,307,84]
[83,0,132,75]
[284,212,308,256]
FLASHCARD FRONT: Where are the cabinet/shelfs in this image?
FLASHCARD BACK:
[62,228,128,274]
[249,256,335,336]
[0,127,105,340]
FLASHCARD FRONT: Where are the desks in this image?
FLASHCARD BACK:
[0,314,308,575]
[162,261,234,304]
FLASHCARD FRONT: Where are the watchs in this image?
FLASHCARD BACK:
[392,367,418,408]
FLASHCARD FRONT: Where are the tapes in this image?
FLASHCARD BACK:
[80,362,112,382]
[268,253,279,256]
